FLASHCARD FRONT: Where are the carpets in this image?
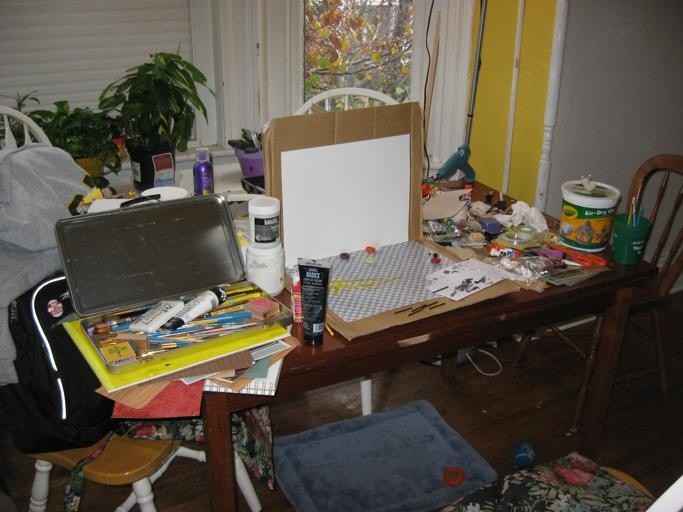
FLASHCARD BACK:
[271,399,497,512]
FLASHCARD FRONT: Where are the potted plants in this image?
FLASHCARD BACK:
[227,127,263,177]
[98,53,215,191]
[43,100,121,178]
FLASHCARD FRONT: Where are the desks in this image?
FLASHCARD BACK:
[202,177,659,511]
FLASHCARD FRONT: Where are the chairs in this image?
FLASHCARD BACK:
[511,154,683,432]
[292,88,428,415]
[0,106,260,512]
[442,453,683,512]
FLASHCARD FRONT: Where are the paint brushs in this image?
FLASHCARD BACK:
[89,283,262,332]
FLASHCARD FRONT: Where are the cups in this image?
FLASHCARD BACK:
[612,213,652,266]
